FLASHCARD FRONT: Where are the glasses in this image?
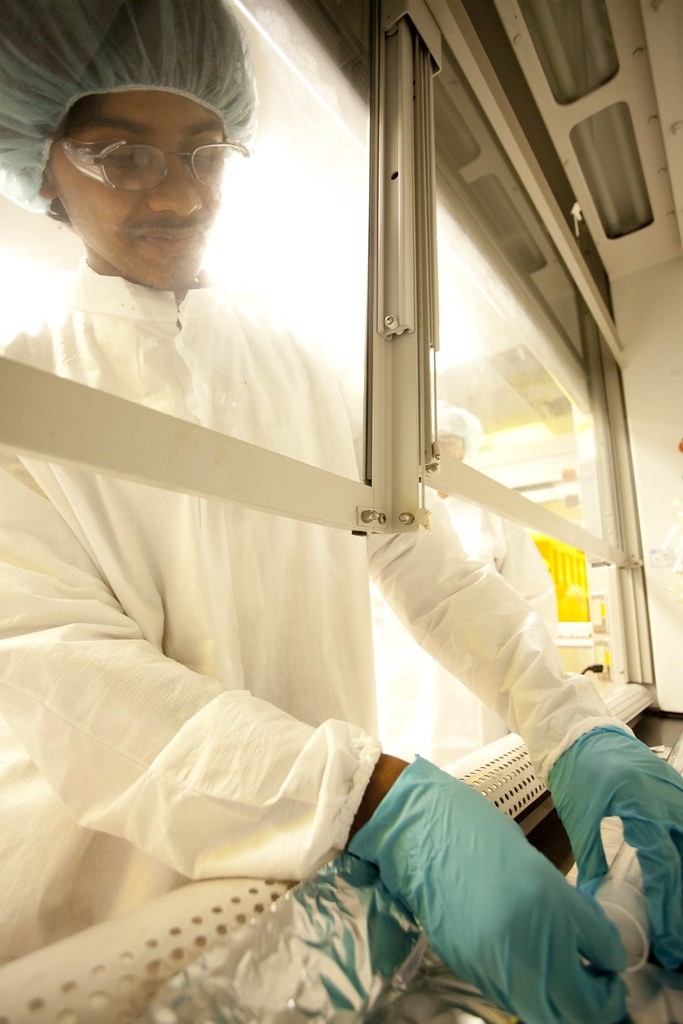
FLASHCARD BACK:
[60,137,251,193]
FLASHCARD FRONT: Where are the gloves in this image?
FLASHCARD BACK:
[347,754,631,1024]
[548,724,683,969]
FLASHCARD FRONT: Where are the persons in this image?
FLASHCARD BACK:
[0,0,683,1024]
